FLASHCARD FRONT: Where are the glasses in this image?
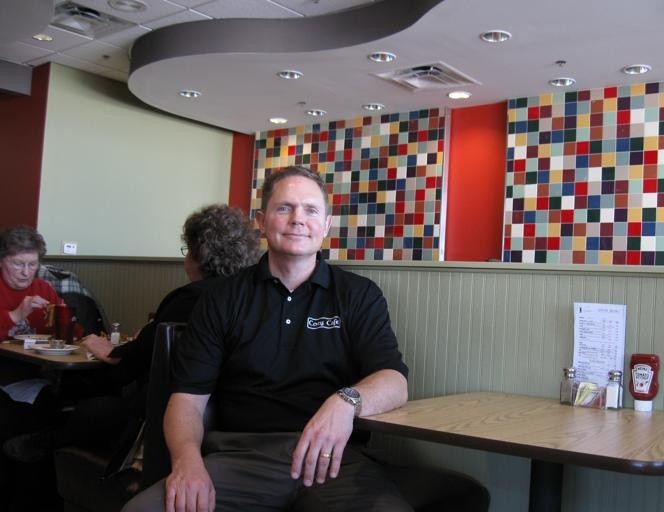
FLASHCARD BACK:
[180,246,190,257]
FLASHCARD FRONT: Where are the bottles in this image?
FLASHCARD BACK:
[111,322,120,345]
[54,303,73,345]
[561,353,660,412]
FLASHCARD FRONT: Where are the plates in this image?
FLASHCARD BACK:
[14,334,79,355]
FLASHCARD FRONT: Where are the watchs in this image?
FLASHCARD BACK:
[336,387,362,419]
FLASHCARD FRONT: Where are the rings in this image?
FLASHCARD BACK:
[320,454,331,458]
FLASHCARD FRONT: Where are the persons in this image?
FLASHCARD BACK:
[121,165,414,512]
[0,204,263,464]
[0,224,85,443]
